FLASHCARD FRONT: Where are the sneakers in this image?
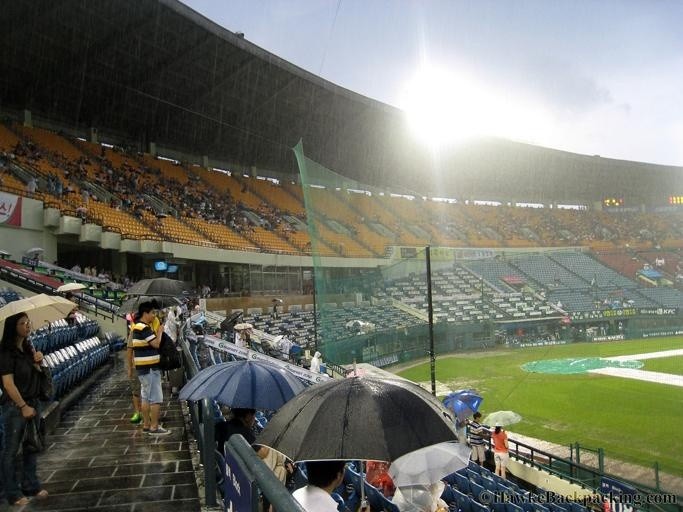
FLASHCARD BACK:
[141,425,171,435]
[130,411,142,422]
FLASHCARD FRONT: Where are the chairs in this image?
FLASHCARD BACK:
[23,313,127,401]
[189,345,589,511]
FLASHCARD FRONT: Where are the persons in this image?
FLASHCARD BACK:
[1,118,683,512]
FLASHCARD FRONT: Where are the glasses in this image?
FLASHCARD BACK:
[21,321,31,325]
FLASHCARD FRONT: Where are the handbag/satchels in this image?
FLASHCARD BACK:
[40,366,52,402]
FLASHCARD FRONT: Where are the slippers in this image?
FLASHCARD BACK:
[14,496,32,505]
[36,490,48,496]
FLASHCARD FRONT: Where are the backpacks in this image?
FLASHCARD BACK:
[160,331,181,369]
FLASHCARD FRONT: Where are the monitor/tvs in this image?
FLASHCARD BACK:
[154,259,168,273]
[168,265,178,274]
